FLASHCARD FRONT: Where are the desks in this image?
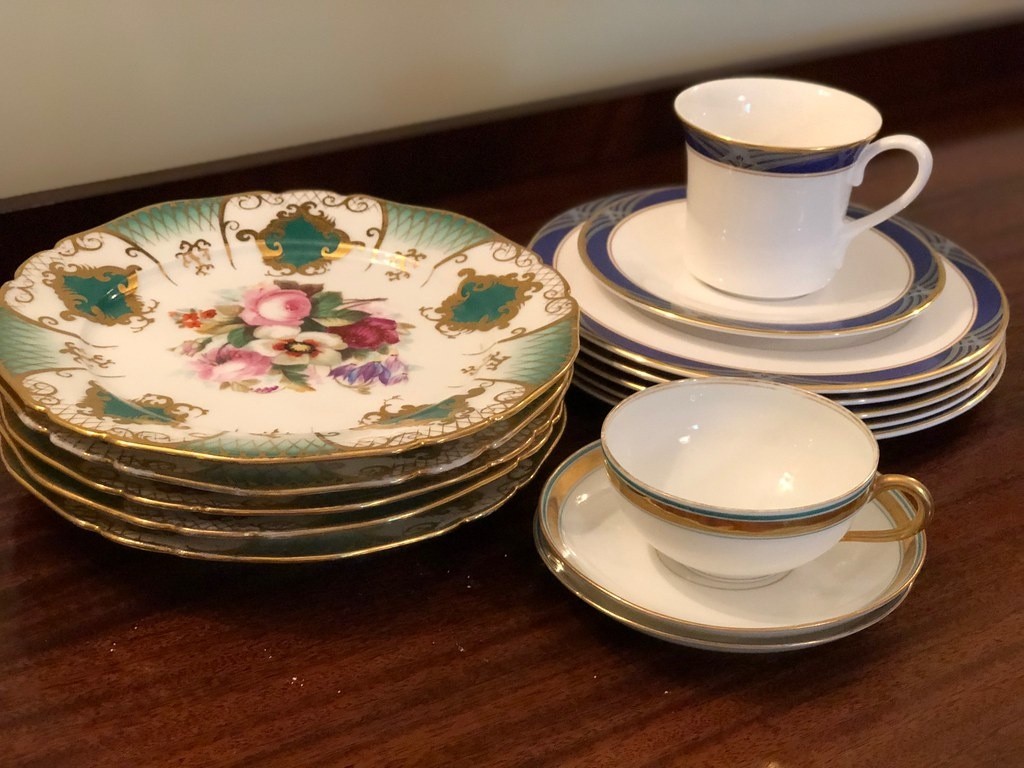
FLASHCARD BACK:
[0,90,1024,768]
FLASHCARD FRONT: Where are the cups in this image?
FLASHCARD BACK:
[672,76,934,300]
[599,377,934,591]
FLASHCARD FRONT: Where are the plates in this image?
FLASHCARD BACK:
[0,190,1010,652]
